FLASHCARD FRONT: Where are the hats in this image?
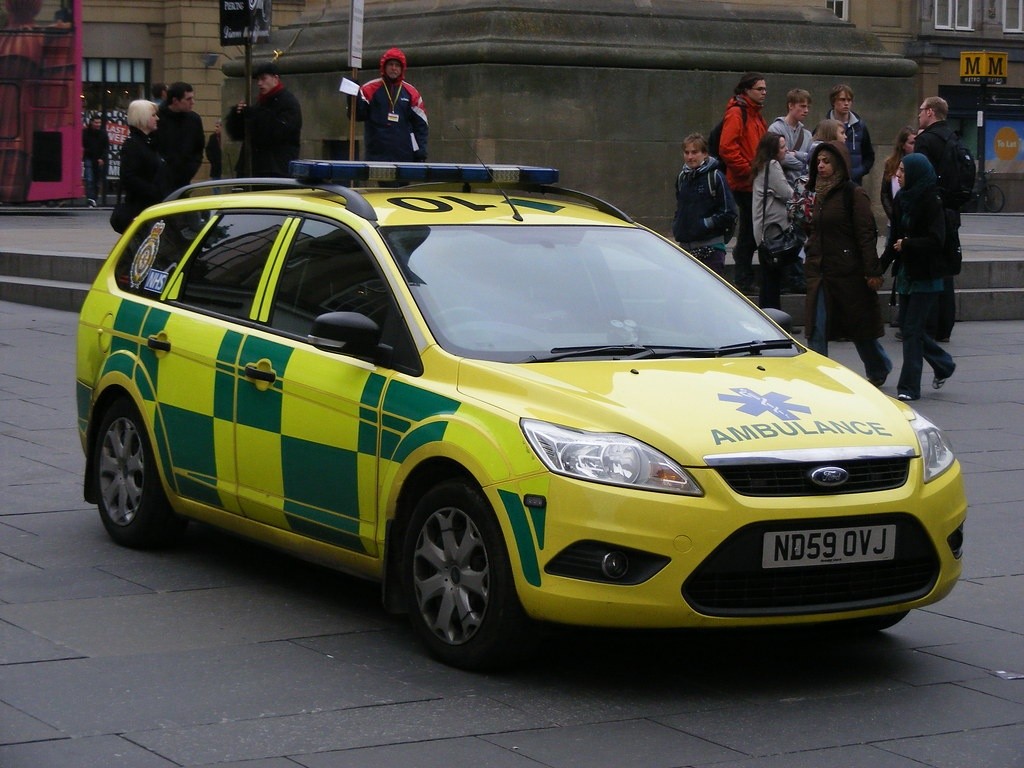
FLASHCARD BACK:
[251,62,280,80]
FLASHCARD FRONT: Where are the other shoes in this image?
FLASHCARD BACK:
[88,197,96,207]
[865,364,892,387]
[895,332,903,339]
[899,392,914,400]
[932,363,956,389]
[890,317,900,328]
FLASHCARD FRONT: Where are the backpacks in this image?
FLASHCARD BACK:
[925,130,975,207]
[678,169,738,244]
[709,104,748,176]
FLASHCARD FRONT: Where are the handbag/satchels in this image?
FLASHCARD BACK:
[758,222,801,273]
[787,173,817,231]
[111,204,149,235]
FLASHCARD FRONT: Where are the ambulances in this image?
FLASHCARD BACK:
[86,156,979,666]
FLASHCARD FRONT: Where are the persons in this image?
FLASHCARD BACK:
[82,116,109,208]
[805,141,893,388]
[149,82,205,199]
[719,73,767,295]
[225,65,301,190]
[768,89,812,293]
[870,153,957,400]
[827,83,876,186]
[803,119,848,174]
[752,133,801,334]
[121,100,174,203]
[347,49,429,162]
[204,120,222,194]
[880,96,962,343]
[672,133,728,275]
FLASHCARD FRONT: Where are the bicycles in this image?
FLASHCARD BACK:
[971,170,1006,214]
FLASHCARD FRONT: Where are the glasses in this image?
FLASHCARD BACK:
[746,87,769,94]
